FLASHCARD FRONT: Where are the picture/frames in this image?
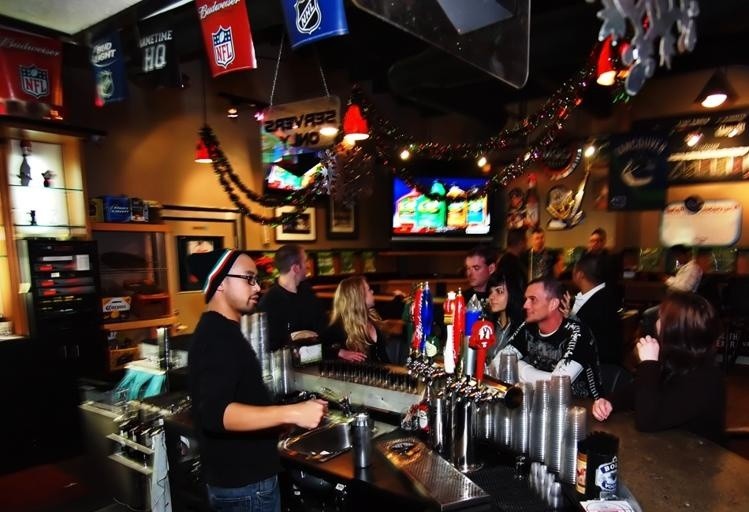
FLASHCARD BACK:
[273,203,317,244]
[325,198,359,240]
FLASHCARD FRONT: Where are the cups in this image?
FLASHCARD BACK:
[587,453,619,500]
[471,350,587,496]
[240,311,295,396]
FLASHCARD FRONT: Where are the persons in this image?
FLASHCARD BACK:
[526,188,538,227]
[592,292,725,444]
[465,228,704,398]
[316,276,390,362]
[505,188,526,229]
[267,245,329,350]
[187,249,328,510]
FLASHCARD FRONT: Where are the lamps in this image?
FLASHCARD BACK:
[595,32,634,86]
[694,68,736,109]
[194,71,221,164]
[342,76,370,142]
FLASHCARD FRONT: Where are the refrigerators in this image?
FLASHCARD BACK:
[16,238,108,378]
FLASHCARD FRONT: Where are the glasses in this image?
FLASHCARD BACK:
[225,273,260,286]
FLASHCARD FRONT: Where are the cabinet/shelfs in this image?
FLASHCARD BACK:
[1,237,112,474]
[0,115,105,337]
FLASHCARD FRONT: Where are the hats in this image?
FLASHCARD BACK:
[188,247,243,304]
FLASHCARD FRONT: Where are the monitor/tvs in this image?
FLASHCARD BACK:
[386,171,503,252]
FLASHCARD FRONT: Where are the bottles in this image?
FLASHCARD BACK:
[525,172,540,228]
[157,327,172,369]
[352,405,372,468]
[119,410,156,465]
